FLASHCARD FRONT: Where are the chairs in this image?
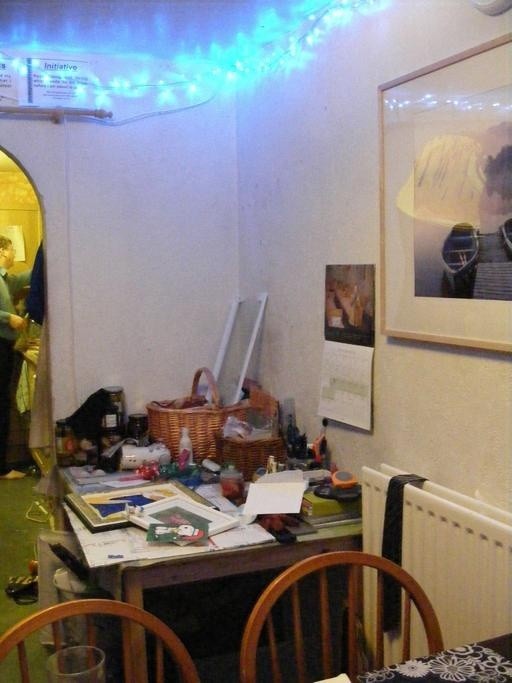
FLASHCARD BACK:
[0,598,200,683]
[239,550,443,683]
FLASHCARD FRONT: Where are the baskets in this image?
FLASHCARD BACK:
[145,366,280,465]
[212,427,288,481]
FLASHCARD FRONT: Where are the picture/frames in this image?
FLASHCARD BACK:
[122,494,240,547]
[376,33,512,353]
[64,480,220,533]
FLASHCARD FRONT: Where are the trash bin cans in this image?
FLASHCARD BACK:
[54,578,151,683]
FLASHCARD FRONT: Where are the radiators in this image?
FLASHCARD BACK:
[360,463,512,665]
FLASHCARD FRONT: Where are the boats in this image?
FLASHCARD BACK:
[440,223,480,289]
[441,270,477,298]
[498,218,511,256]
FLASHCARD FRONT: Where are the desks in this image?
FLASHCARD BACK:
[320,633,512,683]
[61,471,362,682]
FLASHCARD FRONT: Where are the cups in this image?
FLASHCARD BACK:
[220,470,243,501]
[115,444,174,471]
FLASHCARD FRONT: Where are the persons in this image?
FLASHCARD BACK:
[0,234,33,482]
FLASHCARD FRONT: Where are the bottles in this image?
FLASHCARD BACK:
[28,318,39,344]
[180,428,193,465]
[53,384,147,471]
[263,454,286,477]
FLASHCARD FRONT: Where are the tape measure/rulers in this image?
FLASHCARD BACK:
[333,470,358,489]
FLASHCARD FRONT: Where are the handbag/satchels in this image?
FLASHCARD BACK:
[5,576,39,605]
